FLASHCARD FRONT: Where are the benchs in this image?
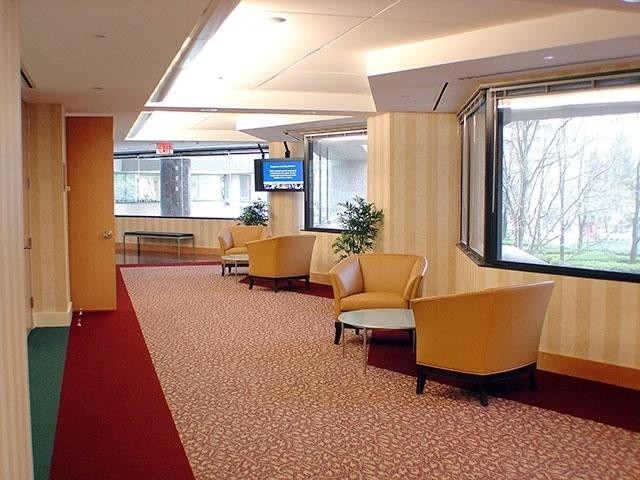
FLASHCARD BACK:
[122,231,195,257]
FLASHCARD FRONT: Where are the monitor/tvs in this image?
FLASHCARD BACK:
[254,159,305,192]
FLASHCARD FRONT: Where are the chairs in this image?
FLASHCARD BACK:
[408,280,557,408]
[328,253,429,345]
[218,224,317,293]
